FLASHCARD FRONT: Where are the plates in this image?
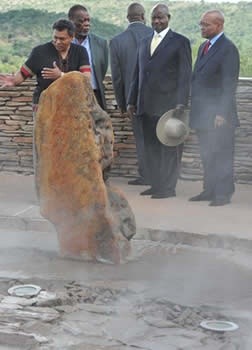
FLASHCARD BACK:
[7,285,41,296]
[200,320,239,331]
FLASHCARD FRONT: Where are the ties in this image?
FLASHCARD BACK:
[151,34,160,50]
[203,41,211,55]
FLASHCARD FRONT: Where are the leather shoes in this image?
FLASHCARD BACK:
[128,179,152,185]
[190,192,214,201]
[209,196,231,206]
[140,188,156,195]
[151,189,176,198]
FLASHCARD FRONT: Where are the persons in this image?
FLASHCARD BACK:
[109,3,153,185]
[189,11,240,206]
[127,5,191,198]
[0,21,91,199]
[68,4,108,110]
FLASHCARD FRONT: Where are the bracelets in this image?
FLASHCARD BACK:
[61,72,64,76]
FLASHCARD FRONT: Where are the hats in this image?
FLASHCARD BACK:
[156,109,190,147]
